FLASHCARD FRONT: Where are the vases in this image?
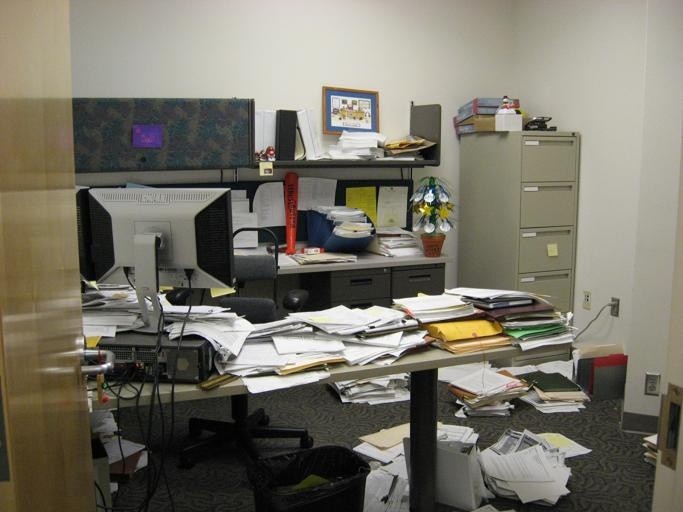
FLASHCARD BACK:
[421,234,445,257]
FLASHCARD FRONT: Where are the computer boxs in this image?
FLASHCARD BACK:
[85,329,217,384]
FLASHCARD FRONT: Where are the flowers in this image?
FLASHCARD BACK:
[406,174,454,233]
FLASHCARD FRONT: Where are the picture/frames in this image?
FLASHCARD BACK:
[319,86,382,138]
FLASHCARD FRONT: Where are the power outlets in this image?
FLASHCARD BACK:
[607,297,619,315]
[580,291,590,309]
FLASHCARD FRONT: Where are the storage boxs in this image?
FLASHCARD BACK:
[571,336,624,397]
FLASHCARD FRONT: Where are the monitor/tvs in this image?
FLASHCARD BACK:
[75,188,237,333]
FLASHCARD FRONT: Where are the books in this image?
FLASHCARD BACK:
[81,274,594,510]
[642,434,658,466]
[293,130,424,265]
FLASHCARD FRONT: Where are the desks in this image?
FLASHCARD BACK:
[229,239,449,309]
[83,323,575,510]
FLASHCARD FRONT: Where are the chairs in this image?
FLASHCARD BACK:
[184,226,319,501]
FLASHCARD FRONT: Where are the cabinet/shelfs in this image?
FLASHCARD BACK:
[457,130,581,337]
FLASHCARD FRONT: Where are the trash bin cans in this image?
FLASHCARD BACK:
[248,445,370,512]
